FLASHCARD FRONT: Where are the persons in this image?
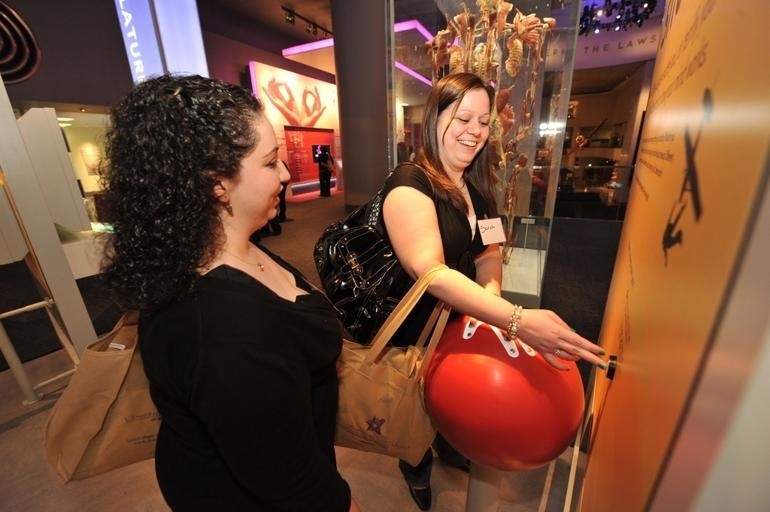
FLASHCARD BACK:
[367,72,608,512]
[100,69,353,512]
[319,146,334,198]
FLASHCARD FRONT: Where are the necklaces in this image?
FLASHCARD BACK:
[224,251,266,272]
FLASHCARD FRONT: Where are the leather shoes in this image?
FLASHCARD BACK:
[408,485,431,510]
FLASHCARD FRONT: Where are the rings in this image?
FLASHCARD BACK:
[555,350,560,356]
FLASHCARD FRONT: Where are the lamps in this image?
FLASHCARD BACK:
[579,0,657,36]
[281,5,333,40]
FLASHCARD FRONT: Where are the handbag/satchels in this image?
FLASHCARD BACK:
[334,264,455,468]
[45,306,162,485]
[312,193,412,345]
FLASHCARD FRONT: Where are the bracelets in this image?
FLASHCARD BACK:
[508,304,521,339]
